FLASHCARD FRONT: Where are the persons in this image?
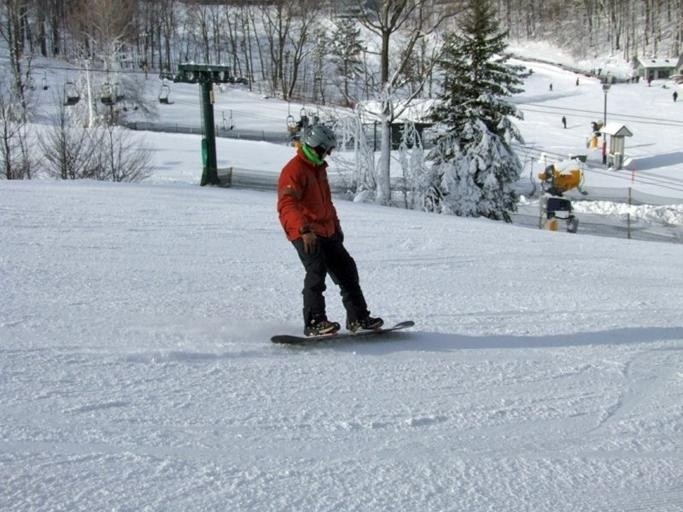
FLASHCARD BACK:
[278,125,384,337]
[562,116,566,128]
[673,91,678,102]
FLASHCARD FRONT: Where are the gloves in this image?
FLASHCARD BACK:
[300,225,321,257]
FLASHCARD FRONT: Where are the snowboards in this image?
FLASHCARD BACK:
[271,321,415,343]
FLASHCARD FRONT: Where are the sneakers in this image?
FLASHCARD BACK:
[345,314,384,332]
[302,318,340,336]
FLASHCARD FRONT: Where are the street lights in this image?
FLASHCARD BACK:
[597,69,614,165]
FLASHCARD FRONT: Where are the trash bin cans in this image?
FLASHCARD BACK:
[614,153,623,170]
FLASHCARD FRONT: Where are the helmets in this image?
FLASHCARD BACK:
[302,123,338,163]
